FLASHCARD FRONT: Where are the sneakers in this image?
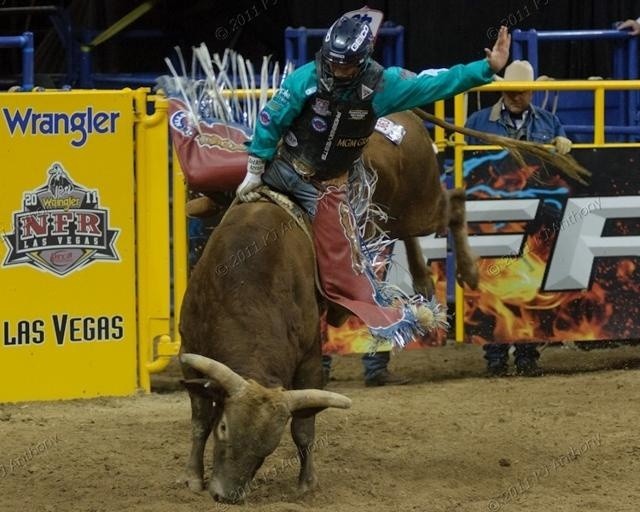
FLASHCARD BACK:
[185,197,223,219]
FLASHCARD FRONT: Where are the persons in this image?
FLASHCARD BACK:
[448,56,574,376]
[322,351,414,388]
[617,17,640,37]
[166,8,513,343]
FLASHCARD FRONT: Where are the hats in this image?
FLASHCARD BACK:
[492,60,549,82]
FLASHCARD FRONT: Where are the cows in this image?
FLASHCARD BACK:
[177,107,593,506]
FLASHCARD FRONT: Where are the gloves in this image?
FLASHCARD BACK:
[236,157,265,202]
[551,136,572,154]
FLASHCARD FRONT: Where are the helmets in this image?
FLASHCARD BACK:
[317,14,374,64]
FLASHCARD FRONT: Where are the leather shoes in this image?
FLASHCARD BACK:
[363,370,410,386]
[484,355,548,375]
